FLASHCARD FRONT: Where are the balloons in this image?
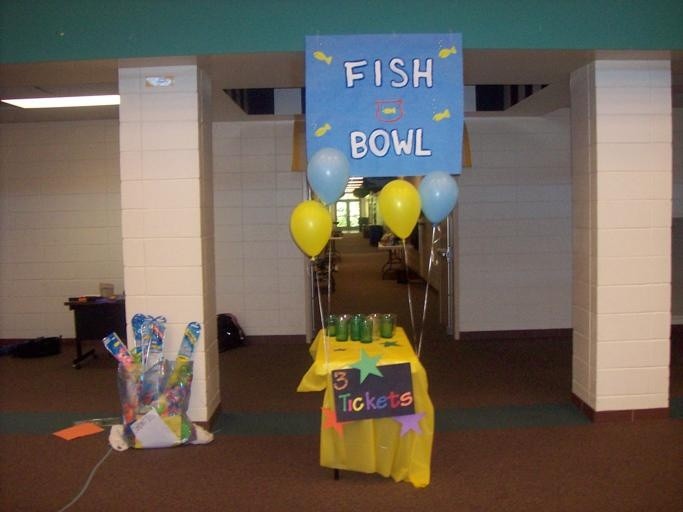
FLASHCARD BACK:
[417,171,459,229]
[379,179,423,242]
[306,147,350,207]
[289,199,334,261]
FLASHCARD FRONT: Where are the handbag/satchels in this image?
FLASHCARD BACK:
[218,314,243,352]
[17,337,61,357]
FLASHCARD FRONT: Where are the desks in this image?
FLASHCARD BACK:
[316,323,426,480]
[64,292,127,371]
[374,235,415,282]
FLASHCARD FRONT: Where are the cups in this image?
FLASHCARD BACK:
[326,313,395,343]
